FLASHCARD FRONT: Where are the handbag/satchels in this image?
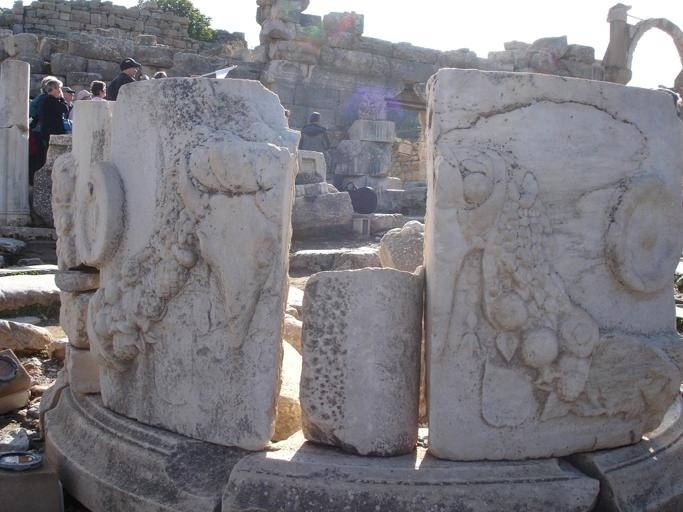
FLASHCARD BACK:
[343,181,377,214]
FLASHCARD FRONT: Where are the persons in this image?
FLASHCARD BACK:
[27,76,106,166]
[153,72,167,78]
[299,112,329,152]
[107,57,141,101]
[137,74,149,81]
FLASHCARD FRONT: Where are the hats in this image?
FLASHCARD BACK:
[120,58,140,70]
